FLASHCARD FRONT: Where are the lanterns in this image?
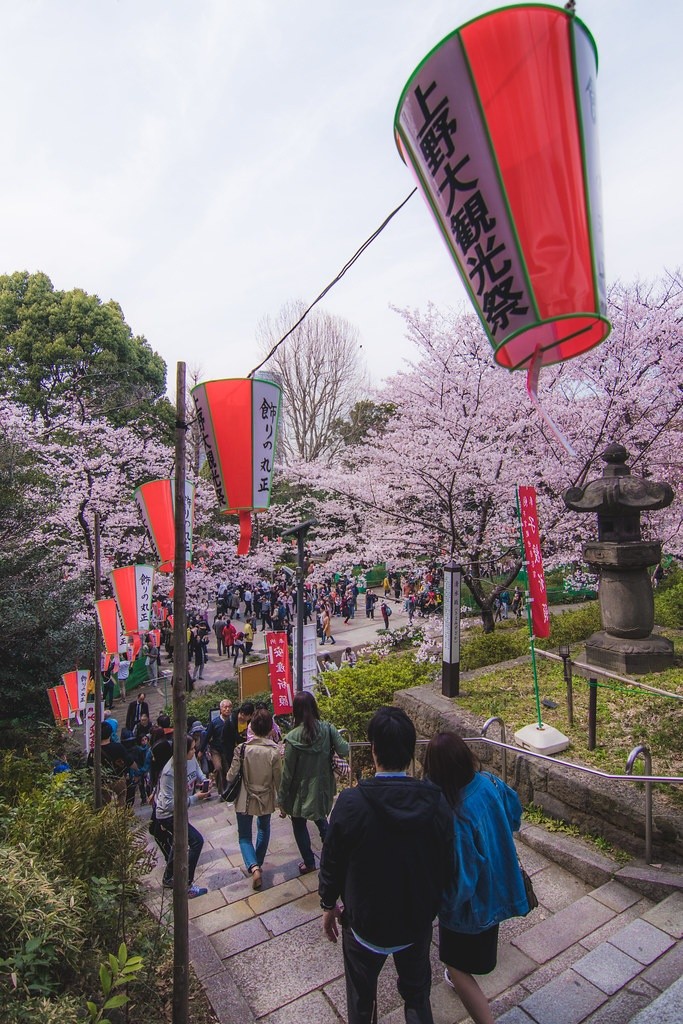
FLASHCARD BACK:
[393,2,611,454]
[148,629,160,646]
[132,480,195,597]
[152,600,173,627]
[190,377,282,554]
[95,565,154,673]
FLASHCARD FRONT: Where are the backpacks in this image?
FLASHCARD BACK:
[385,605,392,616]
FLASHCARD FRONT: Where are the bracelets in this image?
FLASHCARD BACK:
[320,898,336,910]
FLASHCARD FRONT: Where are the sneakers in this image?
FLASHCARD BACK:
[187,885,208,898]
[162,877,174,888]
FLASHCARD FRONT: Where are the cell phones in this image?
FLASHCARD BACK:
[203,781,209,793]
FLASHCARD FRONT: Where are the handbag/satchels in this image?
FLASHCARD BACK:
[521,869,540,908]
[329,723,351,780]
[145,655,150,665]
[220,742,247,802]
[110,675,117,685]
[129,664,134,674]
[201,752,215,773]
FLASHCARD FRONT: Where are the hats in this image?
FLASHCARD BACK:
[189,721,208,735]
[120,729,136,741]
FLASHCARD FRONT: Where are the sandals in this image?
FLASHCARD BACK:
[298,862,311,875]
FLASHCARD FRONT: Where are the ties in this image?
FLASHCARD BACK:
[137,704,140,722]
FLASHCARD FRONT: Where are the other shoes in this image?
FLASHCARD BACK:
[320,643,324,645]
[252,868,264,888]
[445,968,457,987]
[331,641,335,644]
[109,705,114,710]
[230,613,253,620]
[154,683,157,686]
[368,617,374,620]
[198,677,204,680]
[215,648,253,666]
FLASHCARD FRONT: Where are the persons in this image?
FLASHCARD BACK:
[341,647,357,667]
[481,560,511,577]
[156,735,213,898]
[158,557,389,692]
[145,642,158,686]
[323,654,338,672]
[318,707,454,1024]
[279,693,350,873]
[384,560,441,621]
[227,709,282,888]
[423,731,529,1024]
[46,670,91,731]
[191,699,282,801]
[496,586,522,619]
[87,654,130,709]
[88,693,172,808]
